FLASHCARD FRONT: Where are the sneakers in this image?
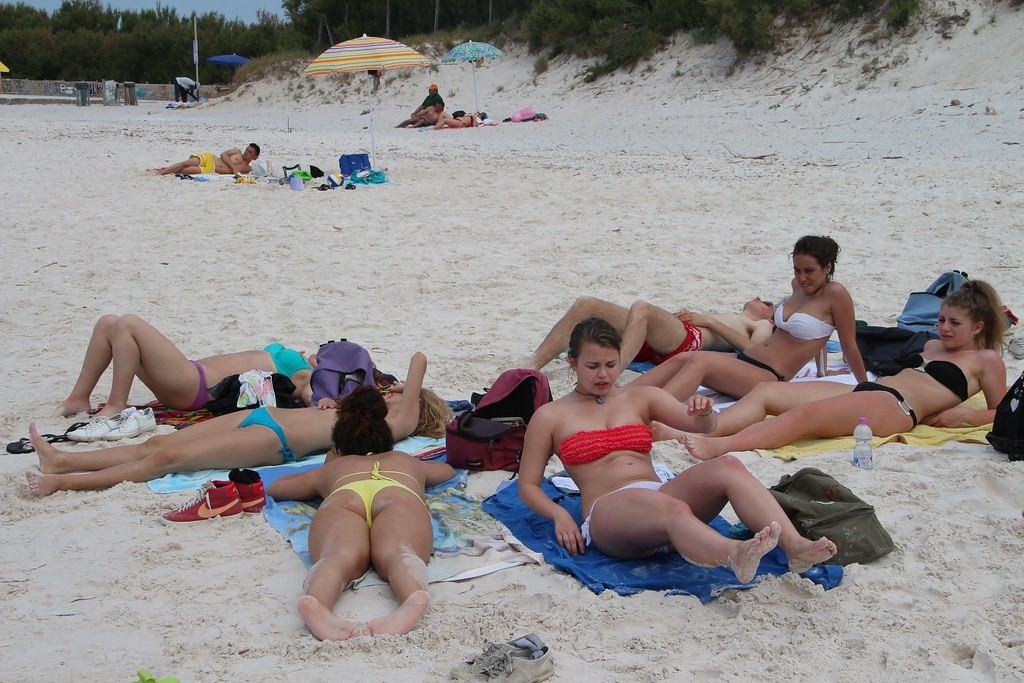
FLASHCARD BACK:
[67,414,123,441]
[229,479,266,513]
[1008,337,1024,359]
[162,480,243,525]
[466,646,556,683]
[449,636,545,680]
[103,407,156,441]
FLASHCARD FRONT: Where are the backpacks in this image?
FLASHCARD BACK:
[769,467,893,567]
[310,337,375,406]
[895,269,971,336]
[445,368,554,471]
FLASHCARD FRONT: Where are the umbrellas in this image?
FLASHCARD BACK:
[441,40,504,113]
[302,33,431,171]
[207,54,250,76]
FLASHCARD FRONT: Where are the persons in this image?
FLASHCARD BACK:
[411,84,445,118]
[156,142,260,176]
[55,314,318,423]
[175,77,200,103]
[649,280,1007,461]
[25,352,453,495]
[394,101,444,128]
[431,111,488,130]
[488,295,778,390]
[617,235,868,403]
[267,385,457,642]
[519,318,839,584]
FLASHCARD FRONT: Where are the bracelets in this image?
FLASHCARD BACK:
[697,409,712,417]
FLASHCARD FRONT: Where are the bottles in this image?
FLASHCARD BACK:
[853,417,873,471]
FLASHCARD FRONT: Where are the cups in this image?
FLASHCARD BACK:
[290,178,302,190]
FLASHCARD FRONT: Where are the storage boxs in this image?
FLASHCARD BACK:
[339,153,371,174]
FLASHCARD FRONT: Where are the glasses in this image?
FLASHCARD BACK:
[764,300,775,316]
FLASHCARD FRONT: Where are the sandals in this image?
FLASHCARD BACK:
[6,433,65,453]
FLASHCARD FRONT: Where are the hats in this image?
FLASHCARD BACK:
[427,84,437,89]
[196,82,200,88]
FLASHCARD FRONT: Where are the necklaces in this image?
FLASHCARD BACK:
[574,388,604,404]
